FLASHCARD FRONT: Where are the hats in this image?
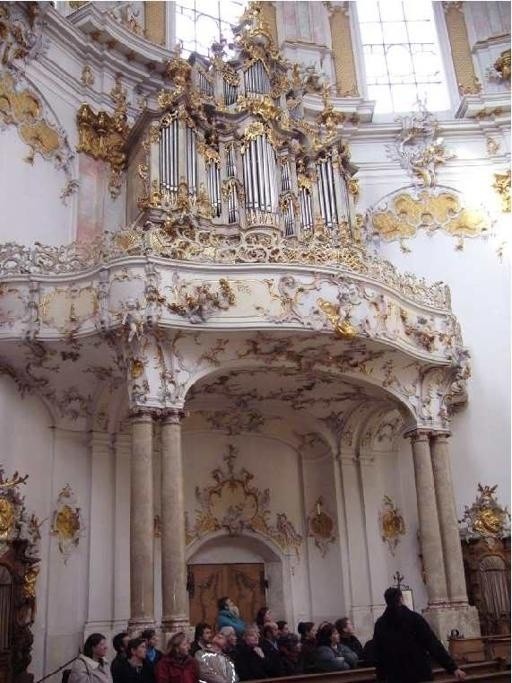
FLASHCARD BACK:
[167,631,186,651]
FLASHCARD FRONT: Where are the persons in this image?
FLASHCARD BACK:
[66,597,363,683]
[372,587,467,683]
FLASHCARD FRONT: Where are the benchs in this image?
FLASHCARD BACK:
[258,655,511,682]
